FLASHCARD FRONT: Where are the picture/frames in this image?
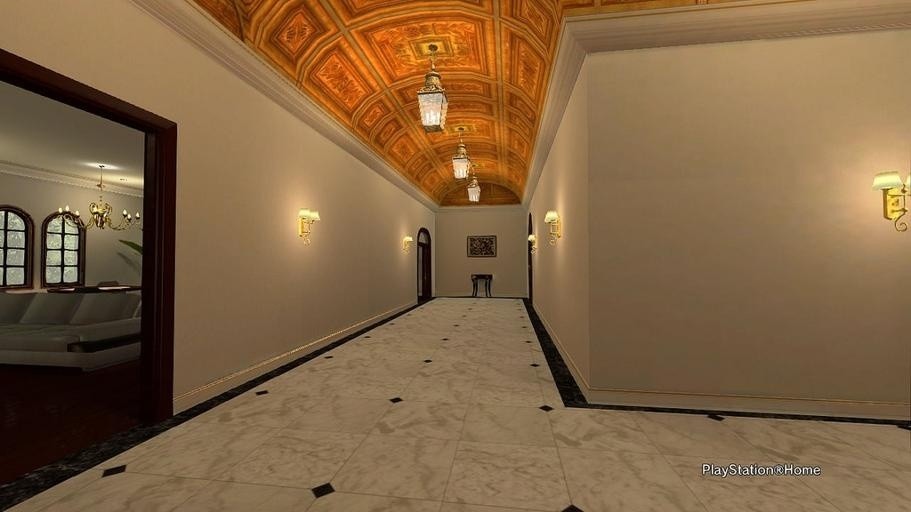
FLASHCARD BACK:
[467,235,498,257]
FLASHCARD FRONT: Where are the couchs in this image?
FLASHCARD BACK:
[1,290,143,373]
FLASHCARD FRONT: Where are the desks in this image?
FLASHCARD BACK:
[471,272,494,297]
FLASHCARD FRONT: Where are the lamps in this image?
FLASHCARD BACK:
[54,163,142,232]
[873,168,911,232]
[417,43,450,135]
[450,125,483,202]
[544,209,563,248]
[298,207,321,247]
